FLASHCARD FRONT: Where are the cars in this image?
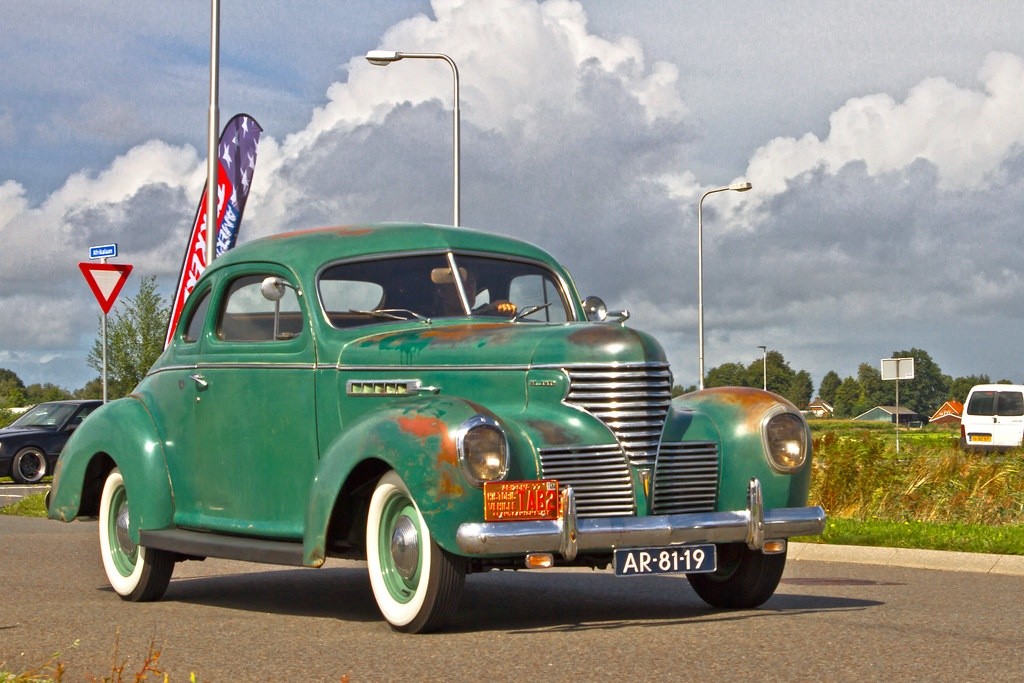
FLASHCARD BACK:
[42,225,826,635]
[0,398,118,486]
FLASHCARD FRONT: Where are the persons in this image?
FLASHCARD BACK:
[442,265,517,316]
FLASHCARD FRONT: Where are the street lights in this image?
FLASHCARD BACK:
[698,182,751,390]
[363,49,463,229]
[757,345,767,392]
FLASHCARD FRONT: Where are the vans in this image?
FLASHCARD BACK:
[958,383,1024,455]
